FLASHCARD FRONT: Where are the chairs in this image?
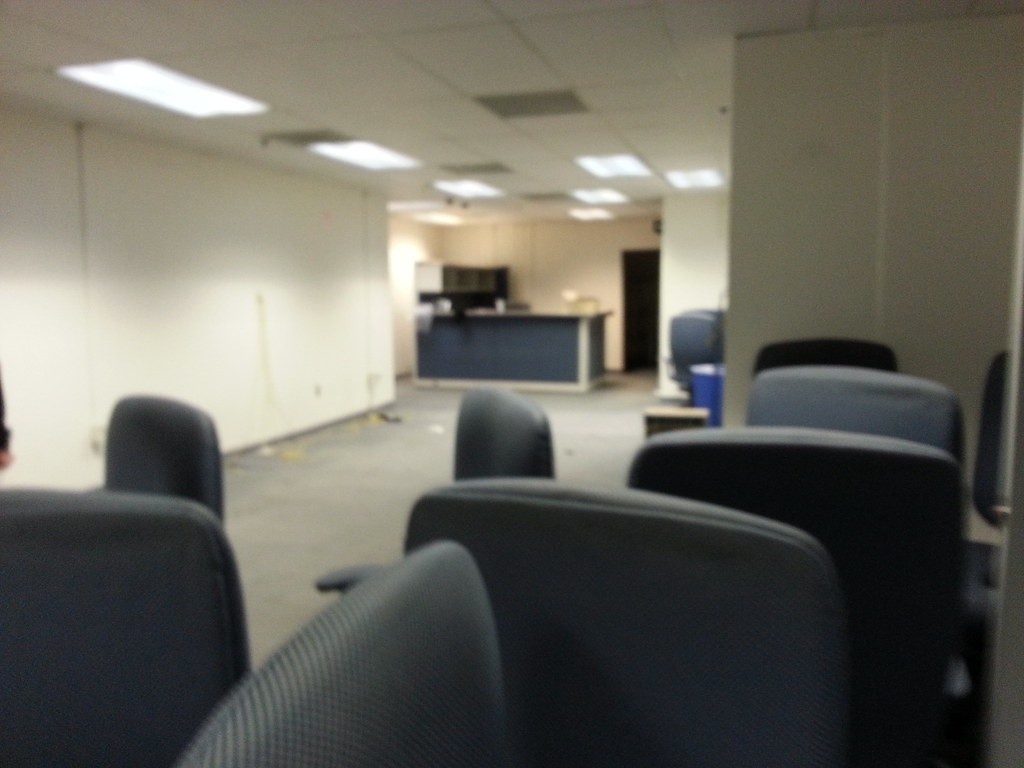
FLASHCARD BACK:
[1,313,1012,768]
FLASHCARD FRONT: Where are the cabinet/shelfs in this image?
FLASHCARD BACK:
[414,262,510,312]
